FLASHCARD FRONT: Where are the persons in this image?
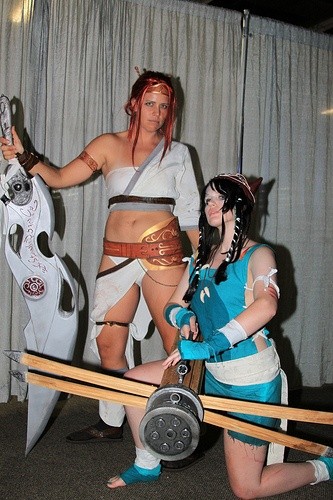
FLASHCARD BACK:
[1,67,205,472]
[106,172,332,500]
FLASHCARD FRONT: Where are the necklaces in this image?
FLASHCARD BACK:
[199,237,250,304]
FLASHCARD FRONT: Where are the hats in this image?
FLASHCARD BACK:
[215,172,263,202]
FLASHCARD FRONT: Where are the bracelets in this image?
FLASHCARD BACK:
[15,150,40,172]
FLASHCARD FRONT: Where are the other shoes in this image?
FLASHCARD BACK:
[65,419,123,442]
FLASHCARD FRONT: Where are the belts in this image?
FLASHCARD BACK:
[103,239,183,259]
